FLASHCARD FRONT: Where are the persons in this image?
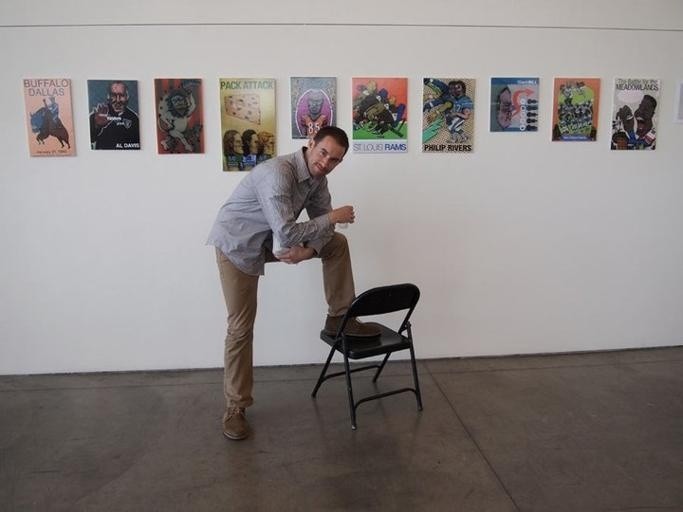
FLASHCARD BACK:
[89,80,138,149]
[203,126,381,440]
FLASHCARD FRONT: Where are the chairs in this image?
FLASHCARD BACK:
[311,283,423,430]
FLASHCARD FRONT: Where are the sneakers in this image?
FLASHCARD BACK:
[222,407,251,440]
[324,313,382,337]
